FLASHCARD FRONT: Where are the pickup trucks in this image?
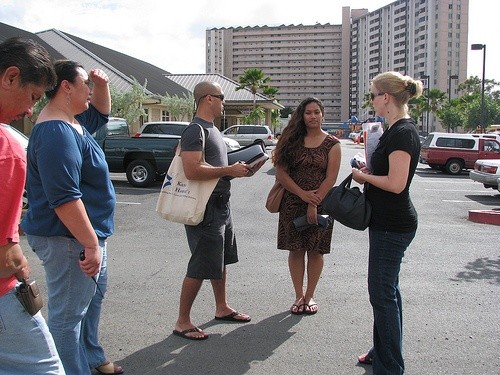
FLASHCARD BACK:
[90,117,182,188]
[419,131,500,174]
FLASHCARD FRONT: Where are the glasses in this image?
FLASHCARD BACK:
[370,92,386,101]
[198,95,225,102]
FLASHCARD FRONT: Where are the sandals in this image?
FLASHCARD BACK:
[94,360,125,375]
[358,354,372,365]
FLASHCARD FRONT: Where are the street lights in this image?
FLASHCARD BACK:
[470,43,486,135]
[420,75,429,137]
[448,75,459,133]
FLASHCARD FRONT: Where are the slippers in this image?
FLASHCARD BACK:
[173,326,208,340]
[215,310,251,322]
[291,299,318,315]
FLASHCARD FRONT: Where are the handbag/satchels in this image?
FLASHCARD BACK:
[155,123,221,226]
[265,177,286,214]
[321,172,372,231]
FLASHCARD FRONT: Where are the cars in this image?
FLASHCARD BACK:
[348,130,363,143]
[469,159,500,193]
[132,121,240,154]
[222,125,278,148]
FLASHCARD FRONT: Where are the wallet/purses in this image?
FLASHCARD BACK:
[16,278,44,317]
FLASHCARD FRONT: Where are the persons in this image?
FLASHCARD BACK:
[0,38,59,375]
[274,97,342,316]
[351,71,423,375]
[21,59,125,375]
[173,81,254,341]
